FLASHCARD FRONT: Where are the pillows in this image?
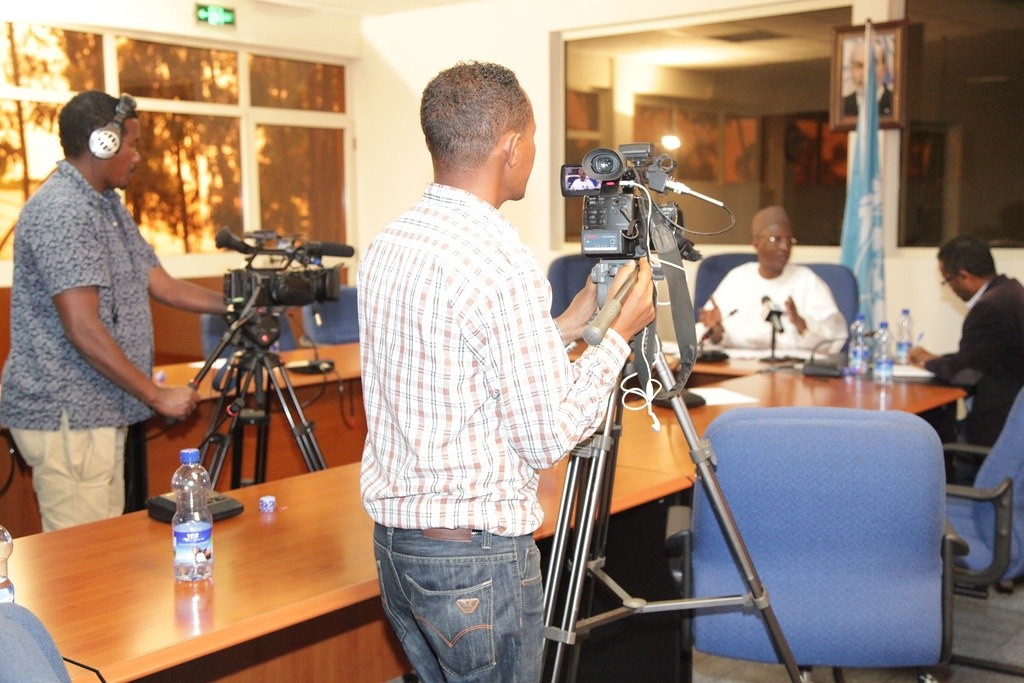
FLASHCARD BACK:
[694,254,863,351]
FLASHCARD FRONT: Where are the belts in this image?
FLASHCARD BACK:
[471,528,535,538]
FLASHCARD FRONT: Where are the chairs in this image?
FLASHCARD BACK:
[549,253,639,320]
[941,388,1023,599]
[301,286,360,344]
[201,310,296,361]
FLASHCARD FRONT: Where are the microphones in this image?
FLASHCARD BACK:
[304,240,355,258]
[761,296,784,333]
[803,332,876,378]
[146,398,245,523]
[697,309,738,362]
[285,313,334,375]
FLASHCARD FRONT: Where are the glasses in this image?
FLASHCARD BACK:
[941,274,958,286]
[758,235,797,247]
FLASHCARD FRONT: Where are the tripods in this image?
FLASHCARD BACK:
[165,310,328,492]
[542,253,803,683]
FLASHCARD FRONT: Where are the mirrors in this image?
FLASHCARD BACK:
[549,0,1024,256]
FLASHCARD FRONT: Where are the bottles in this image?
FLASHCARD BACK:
[872,321,894,385]
[896,309,913,364]
[172,448,213,582]
[848,314,869,373]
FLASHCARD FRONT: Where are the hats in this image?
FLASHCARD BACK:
[752,206,791,239]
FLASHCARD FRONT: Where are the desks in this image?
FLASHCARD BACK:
[627,351,801,390]
[665,407,970,683]
[2,344,371,538]
[9,453,692,683]
[616,368,969,485]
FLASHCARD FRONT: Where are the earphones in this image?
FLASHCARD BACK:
[650,412,661,432]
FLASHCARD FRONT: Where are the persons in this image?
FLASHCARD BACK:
[0,89,235,533]
[692,206,848,355]
[358,60,656,683]
[0,602,72,683]
[909,234,1024,488]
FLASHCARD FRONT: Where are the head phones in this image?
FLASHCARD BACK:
[88,93,136,159]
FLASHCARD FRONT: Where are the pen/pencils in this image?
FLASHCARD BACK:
[156,370,165,382]
[913,331,924,347]
[722,310,738,322]
[757,365,793,373]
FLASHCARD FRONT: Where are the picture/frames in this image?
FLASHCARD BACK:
[829,19,911,129]
